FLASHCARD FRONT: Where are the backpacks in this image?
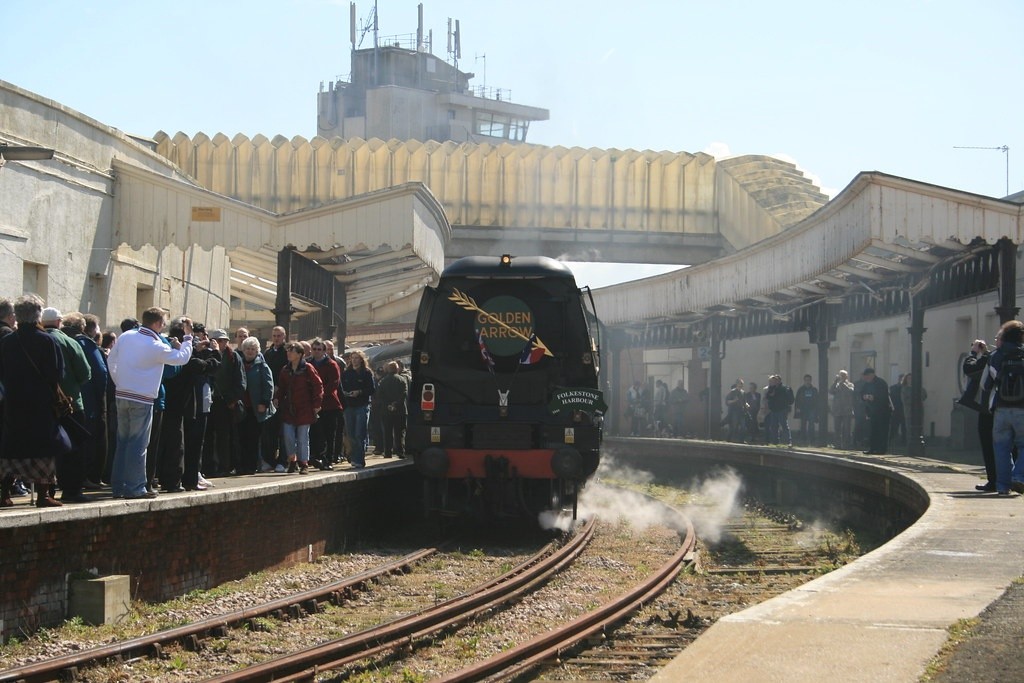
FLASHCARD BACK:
[995,349,1024,403]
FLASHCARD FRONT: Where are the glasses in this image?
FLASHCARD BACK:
[313,348,322,351]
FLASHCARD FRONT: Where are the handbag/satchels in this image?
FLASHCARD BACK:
[54,381,74,418]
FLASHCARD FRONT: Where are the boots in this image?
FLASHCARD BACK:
[0,477,15,507]
[37,483,63,507]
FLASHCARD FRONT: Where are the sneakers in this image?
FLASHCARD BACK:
[10,483,30,497]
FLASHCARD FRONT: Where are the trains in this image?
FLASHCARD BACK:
[406,252,608,522]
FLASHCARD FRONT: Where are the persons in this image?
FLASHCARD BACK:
[0,293,413,509]
[605,320,1024,497]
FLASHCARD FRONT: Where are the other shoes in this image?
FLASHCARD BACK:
[113,472,213,499]
[350,461,363,467]
[1011,481,1024,494]
[61,492,92,504]
[863,450,882,455]
[399,448,406,459]
[313,459,324,471]
[999,491,1009,496]
[77,478,100,490]
[274,464,287,473]
[300,464,309,474]
[287,461,299,473]
[975,482,996,492]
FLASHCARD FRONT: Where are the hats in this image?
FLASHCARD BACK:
[42,308,63,321]
[208,329,231,341]
[863,368,875,375]
